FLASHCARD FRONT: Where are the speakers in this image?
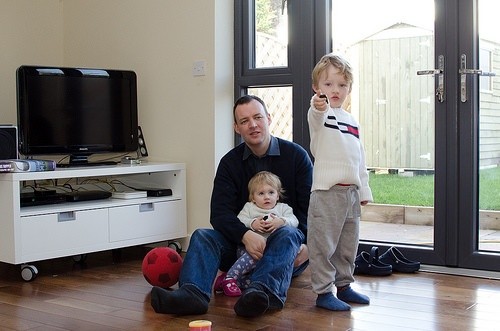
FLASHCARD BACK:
[136,125,148,159]
[0,125,18,160]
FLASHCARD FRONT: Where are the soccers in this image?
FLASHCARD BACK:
[142,246,182,287]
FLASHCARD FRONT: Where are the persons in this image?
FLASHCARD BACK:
[213,171,299,295]
[151,93,313,318]
[307,53,373,310]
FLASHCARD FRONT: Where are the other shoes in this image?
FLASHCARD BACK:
[214,275,224,291]
[222,276,241,296]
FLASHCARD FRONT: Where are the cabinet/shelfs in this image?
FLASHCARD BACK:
[0,161,187,265]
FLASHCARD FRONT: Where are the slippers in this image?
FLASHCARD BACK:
[378,246,420,272]
[354,246,393,275]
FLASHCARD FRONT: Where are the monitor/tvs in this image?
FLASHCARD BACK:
[14,65,138,169]
